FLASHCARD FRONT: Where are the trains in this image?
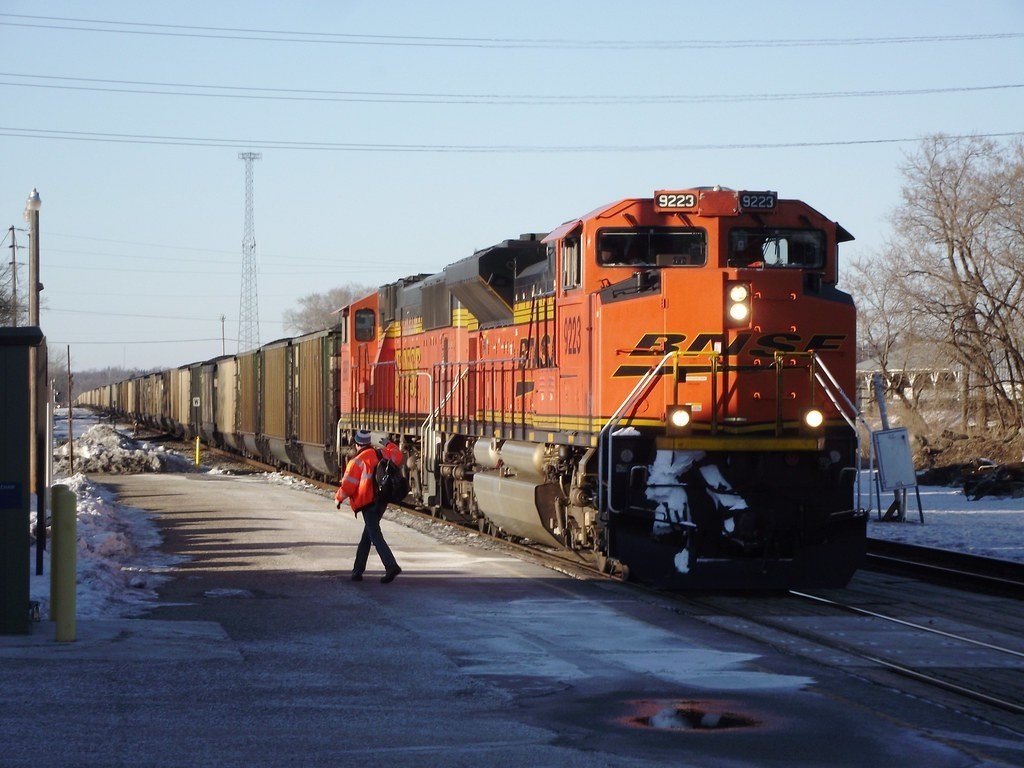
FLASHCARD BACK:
[69,185,875,595]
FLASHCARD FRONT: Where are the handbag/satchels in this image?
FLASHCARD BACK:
[373,449,412,504]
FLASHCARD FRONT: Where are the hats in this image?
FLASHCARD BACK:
[355,430,372,444]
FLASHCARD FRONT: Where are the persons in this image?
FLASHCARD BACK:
[335,430,403,583]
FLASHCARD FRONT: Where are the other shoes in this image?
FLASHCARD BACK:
[350,572,363,581]
[380,566,402,583]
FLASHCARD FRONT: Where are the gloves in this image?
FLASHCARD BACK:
[379,438,390,447]
[334,499,342,510]
